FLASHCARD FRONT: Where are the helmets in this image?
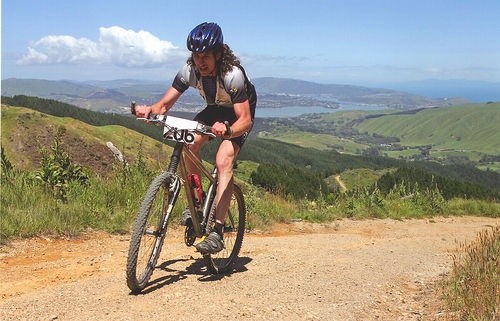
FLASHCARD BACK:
[187,21,223,53]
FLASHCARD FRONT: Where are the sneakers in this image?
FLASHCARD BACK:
[180,210,204,226]
[194,230,226,253]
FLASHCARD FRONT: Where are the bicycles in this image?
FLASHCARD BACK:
[126,100,245,291]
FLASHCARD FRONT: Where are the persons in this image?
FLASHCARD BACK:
[135,22,257,254]
